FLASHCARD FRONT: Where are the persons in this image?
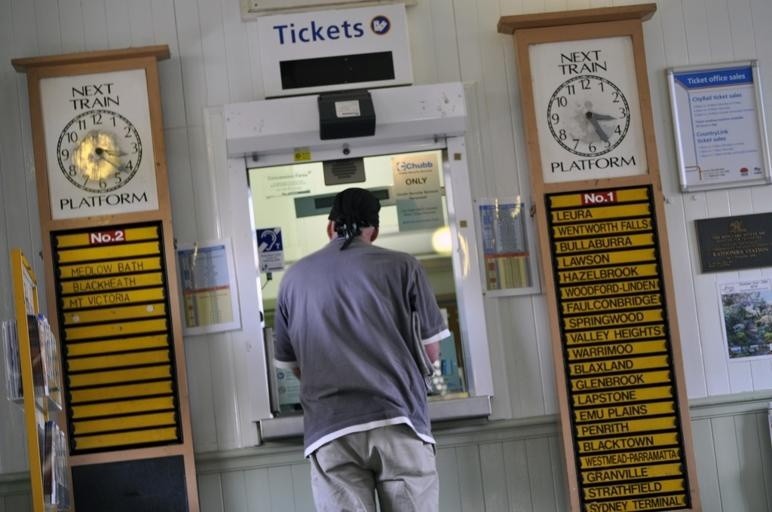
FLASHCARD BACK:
[272,186,452,511]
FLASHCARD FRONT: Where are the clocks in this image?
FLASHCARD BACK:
[545,75,632,160]
[54,109,143,196]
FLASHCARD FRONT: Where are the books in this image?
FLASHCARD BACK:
[1,311,72,510]
